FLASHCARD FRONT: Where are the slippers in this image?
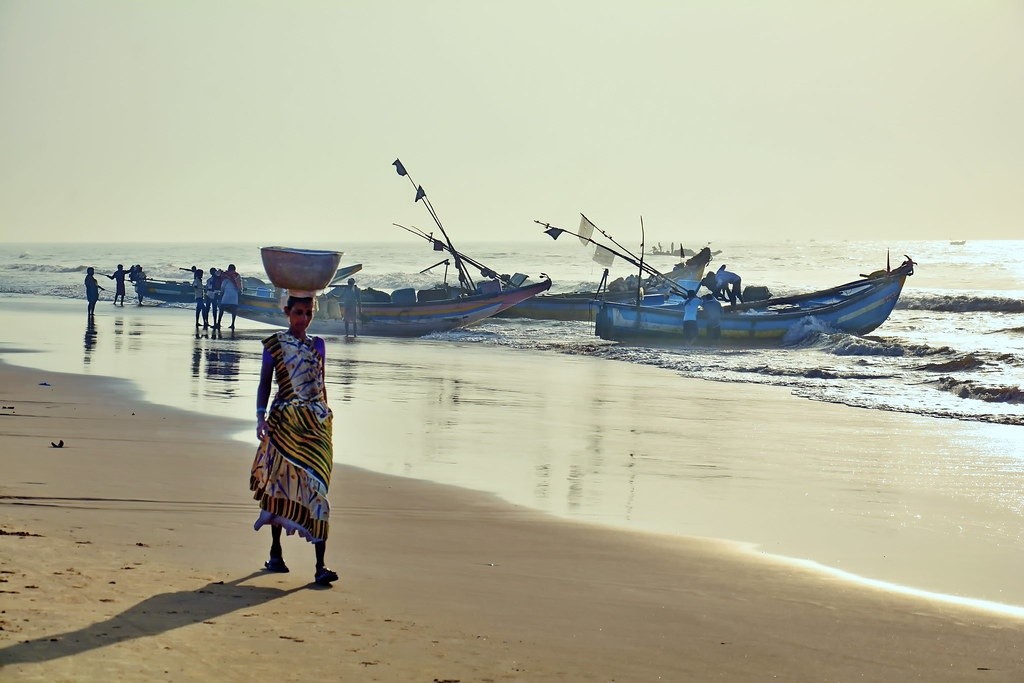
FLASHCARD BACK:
[315,568,338,583]
[265,560,289,572]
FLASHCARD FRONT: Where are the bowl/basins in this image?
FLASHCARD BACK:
[256,245,344,290]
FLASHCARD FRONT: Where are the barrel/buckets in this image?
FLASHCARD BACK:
[257,288,271,297]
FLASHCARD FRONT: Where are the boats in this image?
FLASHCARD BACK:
[203,271,552,335]
[440,247,712,322]
[133,263,363,302]
[589,259,914,342]
[641,251,723,261]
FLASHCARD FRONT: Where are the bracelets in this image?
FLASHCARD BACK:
[256,408,268,418]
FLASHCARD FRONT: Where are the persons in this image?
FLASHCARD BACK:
[701,294,721,339]
[105,265,147,307]
[341,278,363,337]
[702,264,743,313]
[249,288,338,584]
[85,267,105,315]
[684,290,702,343]
[190,264,242,329]
